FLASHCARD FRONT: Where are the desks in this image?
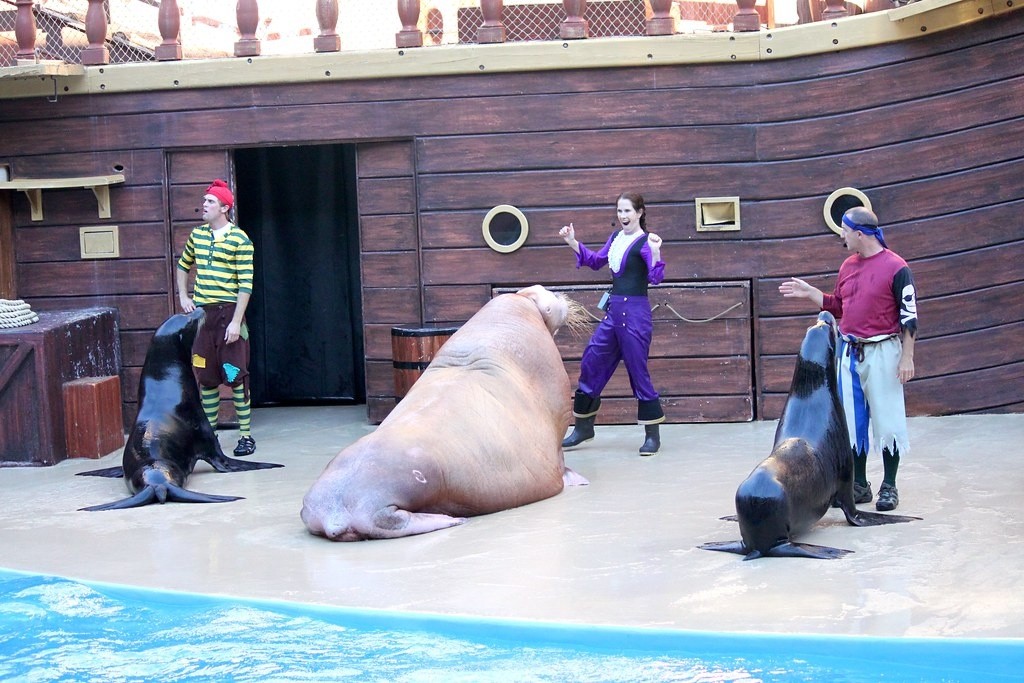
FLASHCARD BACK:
[392,322,463,407]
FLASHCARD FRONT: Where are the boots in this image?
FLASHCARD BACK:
[562,388,601,449]
[637,398,665,456]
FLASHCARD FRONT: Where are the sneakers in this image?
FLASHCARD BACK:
[831,480,872,507]
[234,435,256,456]
[875,481,899,511]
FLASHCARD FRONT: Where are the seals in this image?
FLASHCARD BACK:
[298,284,593,543]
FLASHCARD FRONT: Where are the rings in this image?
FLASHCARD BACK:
[908,376,912,379]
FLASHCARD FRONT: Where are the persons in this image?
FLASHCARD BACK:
[559,192,665,457]
[176,179,257,456]
[779,207,915,510]
